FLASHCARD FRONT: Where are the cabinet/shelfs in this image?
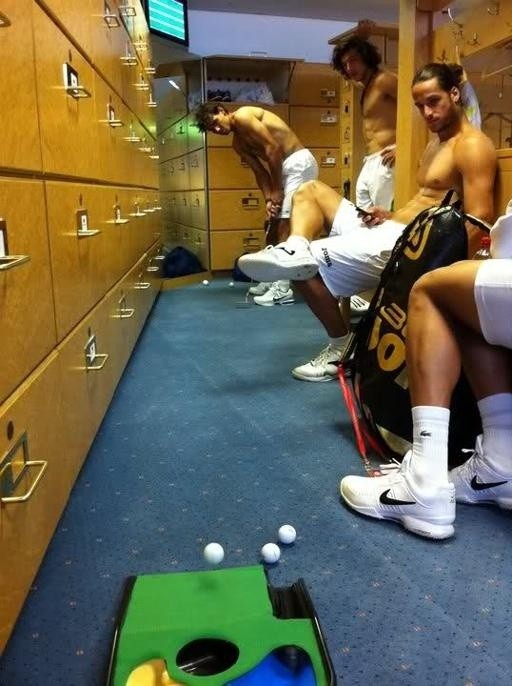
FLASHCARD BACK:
[328,20,399,201]
[428,0,512,218]
[289,62,340,194]
[158,103,289,272]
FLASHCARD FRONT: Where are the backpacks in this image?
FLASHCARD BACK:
[351,191,492,459]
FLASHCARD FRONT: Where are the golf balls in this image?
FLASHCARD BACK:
[204,542,225,563]
[228,282,235,285]
[262,544,280,563]
[202,279,209,288]
[278,525,296,544]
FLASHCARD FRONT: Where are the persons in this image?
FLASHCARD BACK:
[195,102,319,307]
[237,62,497,382]
[333,36,397,211]
[339,200,512,541]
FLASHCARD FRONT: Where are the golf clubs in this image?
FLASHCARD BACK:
[236,221,272,305]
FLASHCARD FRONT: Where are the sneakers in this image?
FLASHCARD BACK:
[340,450,456,538]
[250,281,274,295]
[292,343,355,382]
[254,282,295,306]
[237,242,318,283]
[449,434,512,508]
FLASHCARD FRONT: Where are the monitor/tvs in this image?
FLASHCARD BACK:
[145,0,189,47]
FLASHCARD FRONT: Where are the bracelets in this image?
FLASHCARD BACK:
[265,198,271,204]
